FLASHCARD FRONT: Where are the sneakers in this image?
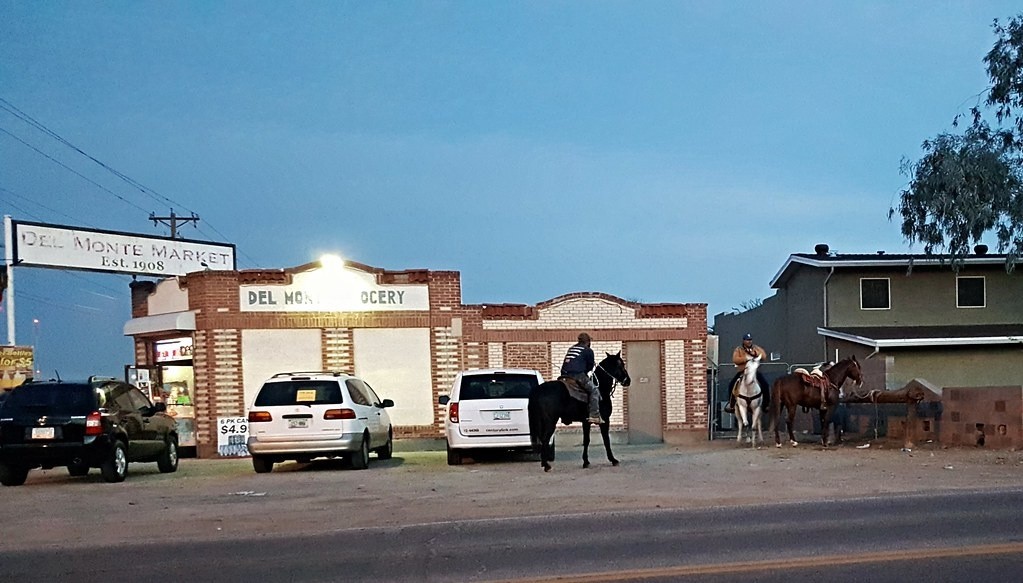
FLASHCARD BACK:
[588,413,605,423]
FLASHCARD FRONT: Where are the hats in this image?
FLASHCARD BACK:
[742,332,752,340]
[578,332,593,341]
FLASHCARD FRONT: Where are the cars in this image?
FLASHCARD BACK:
[438,368,555,466]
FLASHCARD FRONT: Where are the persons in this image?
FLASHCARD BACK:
[560,332,605,423]
[724,332,770,413]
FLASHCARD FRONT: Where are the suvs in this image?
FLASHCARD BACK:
[0,375,180,486]
[246,370,395,474]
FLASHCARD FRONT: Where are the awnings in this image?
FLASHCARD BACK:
[124,311,196,336]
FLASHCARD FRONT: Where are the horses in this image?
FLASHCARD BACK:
[733,353,864,449]
[527,351,631,471]
